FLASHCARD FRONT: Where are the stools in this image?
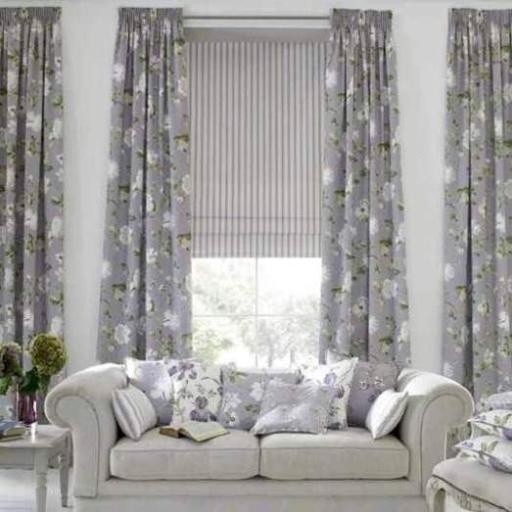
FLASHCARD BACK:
[423,456,512,512]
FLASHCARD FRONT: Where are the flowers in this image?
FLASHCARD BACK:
[0,330,71,395]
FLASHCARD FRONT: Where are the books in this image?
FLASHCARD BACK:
[0,417,29,442]
[158,420,231,443]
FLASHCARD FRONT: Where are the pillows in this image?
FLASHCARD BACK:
[111,384,157,441]
[168,364,224,424]
[293,357,358,431]
[249,380,335,436]
[125,357,172,426]
[219,369,300,430]
[347,363,404,427]
[364,388,407,439]
[452,390,512,473]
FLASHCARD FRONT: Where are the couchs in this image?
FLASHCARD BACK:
[44,360,476,512]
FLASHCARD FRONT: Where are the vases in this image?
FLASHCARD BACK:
[14,385,40,437]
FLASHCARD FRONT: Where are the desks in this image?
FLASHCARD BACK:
[0,423,73,512]
